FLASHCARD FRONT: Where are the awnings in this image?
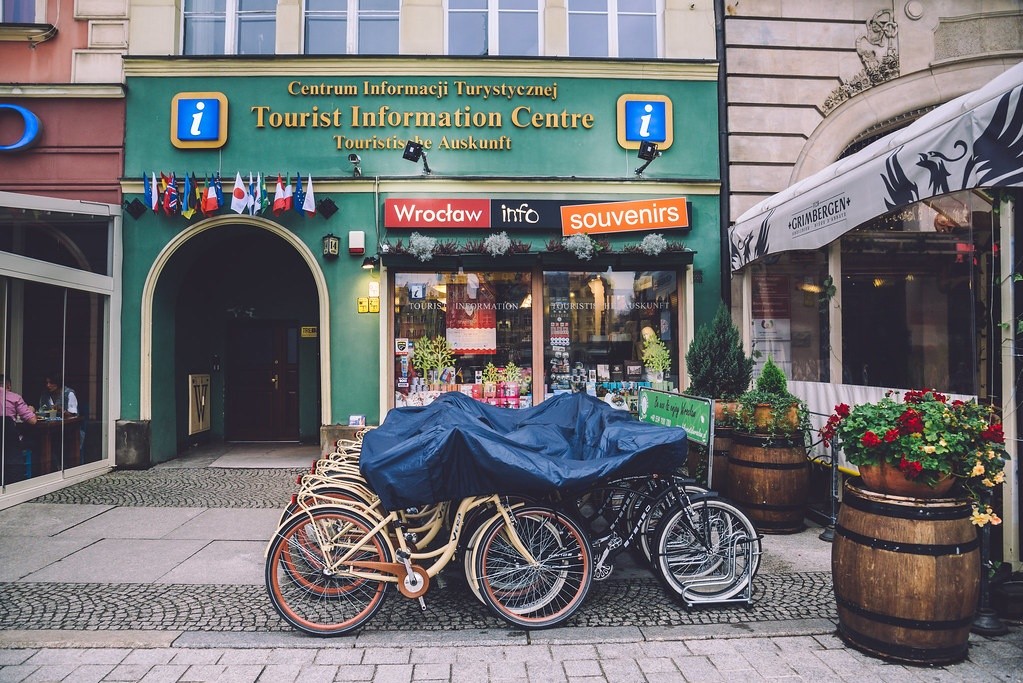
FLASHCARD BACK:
[728,62,1023,273]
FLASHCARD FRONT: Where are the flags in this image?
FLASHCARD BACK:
[142,171,317,220]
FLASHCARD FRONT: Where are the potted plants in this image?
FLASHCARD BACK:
[684,297,754,426]
[735,355,814,432]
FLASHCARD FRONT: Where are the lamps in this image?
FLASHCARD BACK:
[316,196,338,218]
[125,197,147,219]
[361,257,376,270]
[402,140,431,174]
[635,140,662,176]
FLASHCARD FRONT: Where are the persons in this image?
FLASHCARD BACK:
[0,374,37,450]
[37,370,81,418]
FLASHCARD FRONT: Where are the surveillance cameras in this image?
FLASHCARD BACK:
[348,154,361,164]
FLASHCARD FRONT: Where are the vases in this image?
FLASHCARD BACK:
[460,253,539,273]
[858,461,955,498]
[539,251,620,267]
[378,253,460,271]
[620,252,697,266]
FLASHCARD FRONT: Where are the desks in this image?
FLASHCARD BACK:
[12,413,85,475]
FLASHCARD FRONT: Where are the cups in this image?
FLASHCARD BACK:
[423,385,429,391]
[412,377,419,385]
[417,385,422,391]
[580,369,586,376]
[582,376,587,381]
[572,369,577,375]
[49,405,57,418]
[575,362,583,369]
[575,376,580,382]
[420,378,425,385]
[410,385,416,393]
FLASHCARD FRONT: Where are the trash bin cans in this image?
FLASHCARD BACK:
[114,419,151,471]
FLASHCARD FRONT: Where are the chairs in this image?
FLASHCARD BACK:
[0,416,31,485]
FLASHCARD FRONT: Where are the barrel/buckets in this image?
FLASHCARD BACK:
[832,476,982,667]
[728,430,809,535]
[688,425,733,498]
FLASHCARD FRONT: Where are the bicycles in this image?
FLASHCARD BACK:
[265,383,762,638]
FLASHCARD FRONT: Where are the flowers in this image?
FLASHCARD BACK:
[621,233,686,256]
[378,230,461,263]
[461,231,534,256]
[544,232,615,261]
[817,386,1011,527]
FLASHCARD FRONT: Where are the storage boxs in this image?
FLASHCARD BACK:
[391,359,676,417]
